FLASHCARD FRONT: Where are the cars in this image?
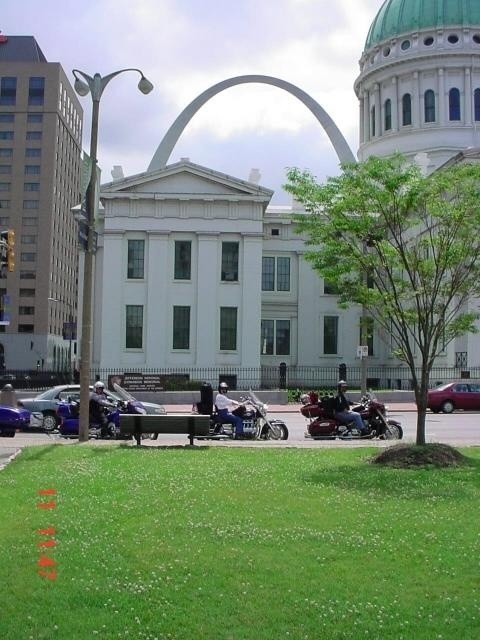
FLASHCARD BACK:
[0,405,31,437]
[425,380,480,413]
[14,411,44,433]
[17,385,167,431]
[1,374,16,380]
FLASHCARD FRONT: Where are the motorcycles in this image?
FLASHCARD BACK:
[300,391,404,440]
[57,383,158,441]
[191,389,288,442]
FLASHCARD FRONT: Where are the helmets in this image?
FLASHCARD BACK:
[338,380,349,387]
[93,381,105,393]
[219,383,229,390]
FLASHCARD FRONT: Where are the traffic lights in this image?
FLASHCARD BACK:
[3,230,15,272]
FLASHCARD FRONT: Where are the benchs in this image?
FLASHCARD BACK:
[118,413,212,446]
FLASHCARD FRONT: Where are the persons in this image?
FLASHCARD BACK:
[331,379,373,436]
[214,380,245,440]
[343,383,360,410]
[89,380,118,434]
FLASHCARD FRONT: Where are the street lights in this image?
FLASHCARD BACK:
[48,297,73,369]
[70,67,155,442]
